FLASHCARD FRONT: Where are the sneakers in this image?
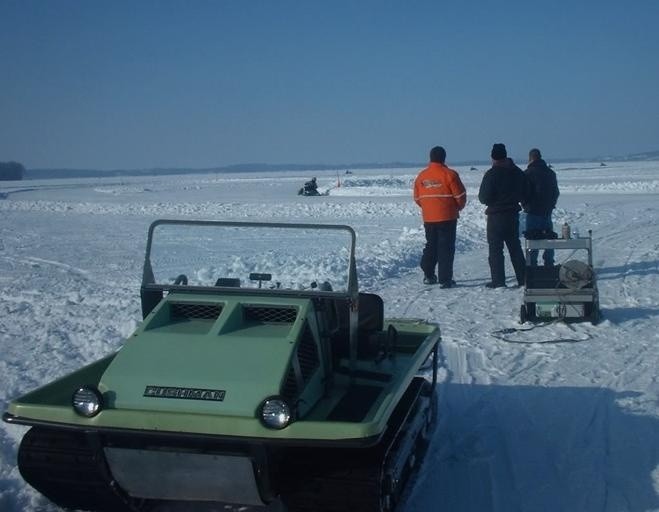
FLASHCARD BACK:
[424,275,455,288]
[487,282,505,288]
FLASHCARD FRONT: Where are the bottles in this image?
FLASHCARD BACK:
[572,227,580,239]
[562,221,570,239]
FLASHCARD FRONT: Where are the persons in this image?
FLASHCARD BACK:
[298,175,319,198]
[412,145,468,291]
[477,140,533,289]
[519,148,561,269]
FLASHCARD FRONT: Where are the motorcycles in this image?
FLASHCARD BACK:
[298,184,322,196]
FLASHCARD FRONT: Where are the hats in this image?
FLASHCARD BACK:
[492,144,506,160]
